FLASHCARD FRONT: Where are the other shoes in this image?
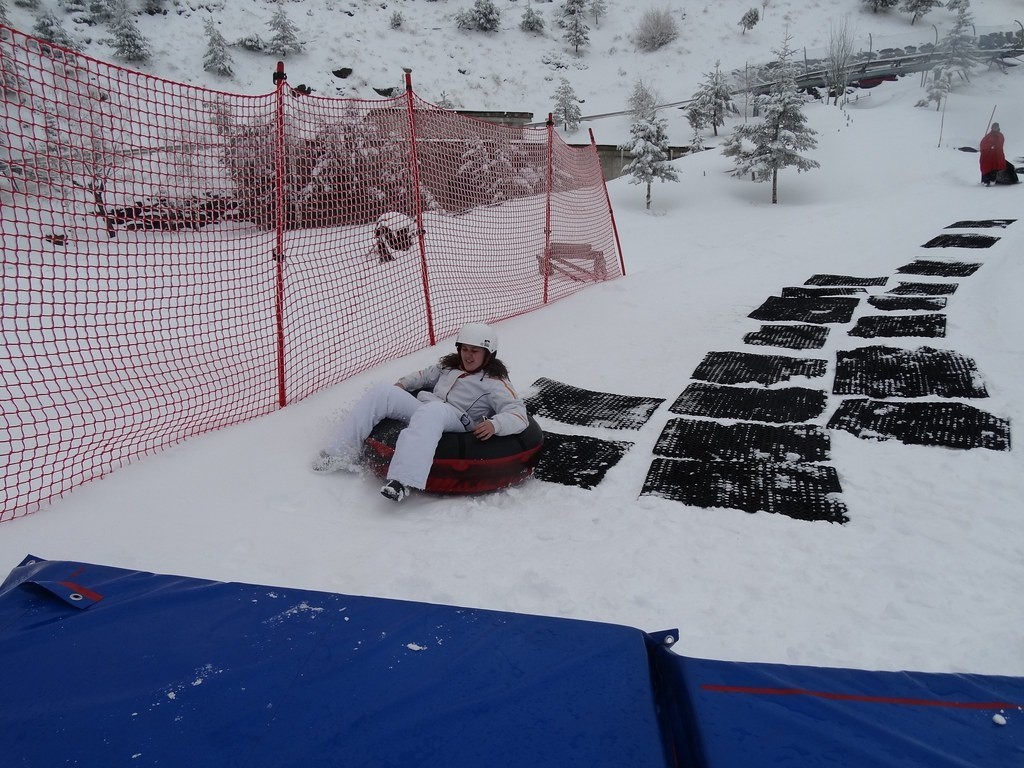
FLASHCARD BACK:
[382,480,407,502]
[312,450,336,470]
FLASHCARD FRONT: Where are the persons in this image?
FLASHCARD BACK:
[314,323,531,501]
[979,123,1006,184]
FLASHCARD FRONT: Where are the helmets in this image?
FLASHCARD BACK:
[455,322,498,354]
